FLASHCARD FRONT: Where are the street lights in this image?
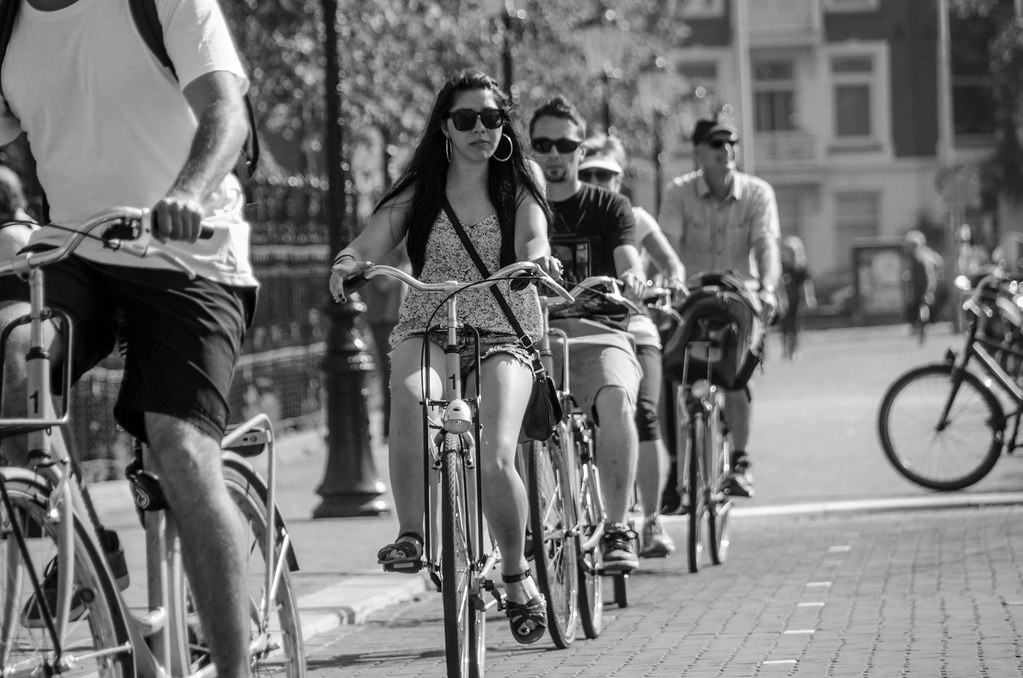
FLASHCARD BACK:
[675,83,719,172]
[634,50,680,222]
[569,1,631,136]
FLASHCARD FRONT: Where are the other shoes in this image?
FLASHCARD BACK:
[662,471,688,517]
[599,522,639,569]
[726,466,753,498]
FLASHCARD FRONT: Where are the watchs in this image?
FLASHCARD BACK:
[763,285,776,294]
[672,274,684,282]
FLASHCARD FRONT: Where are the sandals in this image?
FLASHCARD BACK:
[377,532,424,563]
[502,566,549,642]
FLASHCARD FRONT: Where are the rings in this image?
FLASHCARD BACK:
[557,262,563,269]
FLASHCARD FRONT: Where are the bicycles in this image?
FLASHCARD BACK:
[0,203,311,678]
[332,258,576,678]
[648,272,778,575]
[876,271,1023,493]
[523,270,690,651]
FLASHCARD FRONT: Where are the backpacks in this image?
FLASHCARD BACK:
[660,286,768,403]
[0,0,266,203]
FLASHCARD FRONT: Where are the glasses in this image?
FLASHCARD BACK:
[531,135,584,156]
[578,169,613,184]
[442,108,508,131]
[702,140,739,152]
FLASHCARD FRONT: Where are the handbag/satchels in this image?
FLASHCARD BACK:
[701,268,765,318]
[522,376,563,441]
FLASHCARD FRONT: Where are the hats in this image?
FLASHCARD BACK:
[691,113,739,145]
[578,135,624,175]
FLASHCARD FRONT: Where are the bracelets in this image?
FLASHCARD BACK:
[333,255,355,265]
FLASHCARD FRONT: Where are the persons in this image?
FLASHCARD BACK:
[525,97,647,571]
[657,117,781,495]
[578,135,691,554]
[0,0,260,678]
[903,230,944,329]
[328,72,562,644]
[779,236,816,359]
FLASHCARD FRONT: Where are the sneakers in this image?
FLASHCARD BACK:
[20,527,130,627]
[640,518,674,556]
